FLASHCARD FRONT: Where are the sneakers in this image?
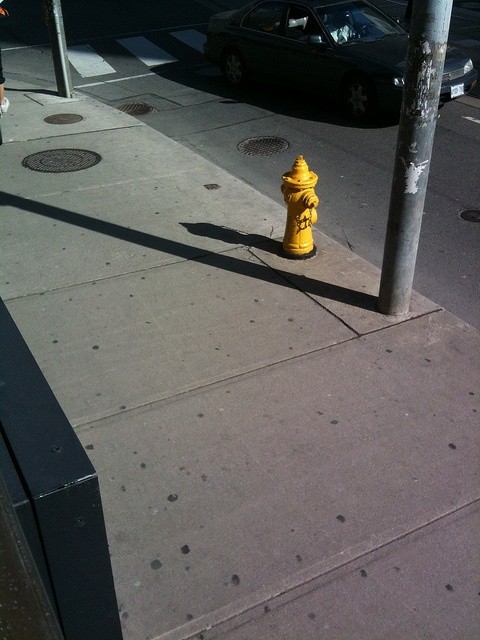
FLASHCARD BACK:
[0,97,9,118]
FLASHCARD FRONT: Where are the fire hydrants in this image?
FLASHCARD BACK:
[281,154,320,260]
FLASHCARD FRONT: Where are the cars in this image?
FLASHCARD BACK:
[203,0,478,126]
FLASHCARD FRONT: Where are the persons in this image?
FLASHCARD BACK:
[0,48,10,116]
[324,13,359,44]
